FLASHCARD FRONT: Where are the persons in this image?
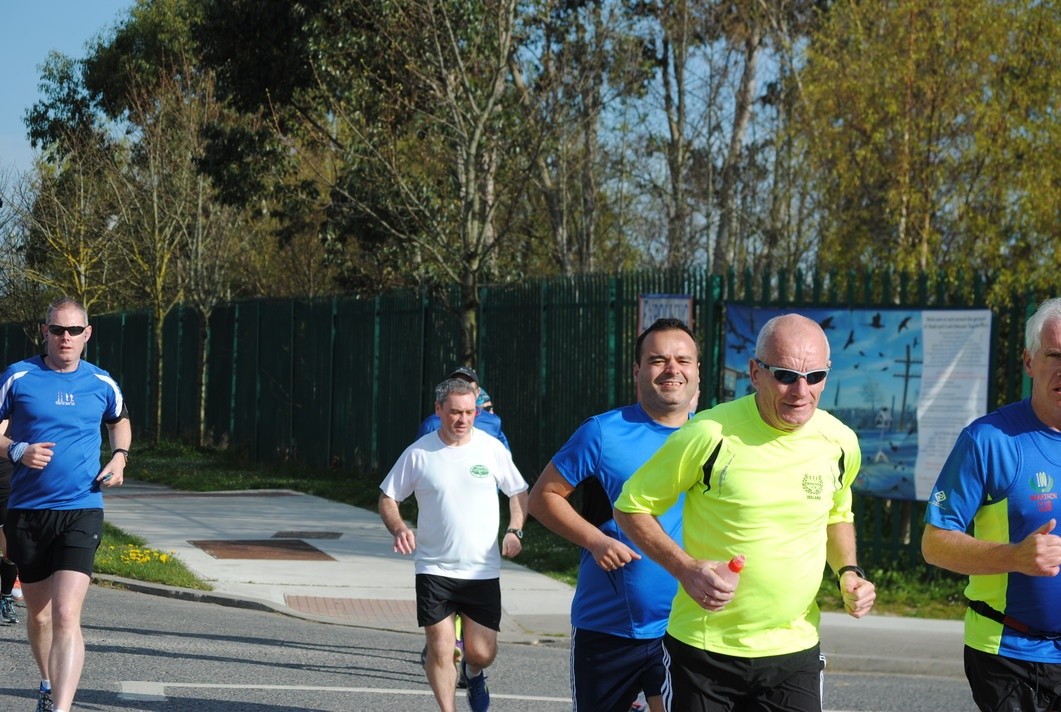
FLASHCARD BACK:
[377,379,529,712]
[418,366,510,687]
[0,299,133,712]
[920,298,1061,712]
[612,313,876,711]
[0,418,23,626]
[528,319,698,712]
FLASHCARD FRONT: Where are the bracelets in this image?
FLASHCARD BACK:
[7,442,29,462]
[835,565,866,592]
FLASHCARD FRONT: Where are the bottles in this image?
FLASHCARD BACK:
[714,555,745,588]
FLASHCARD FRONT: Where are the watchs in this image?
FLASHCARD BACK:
[506,528,523,539]
[112,449,129,464]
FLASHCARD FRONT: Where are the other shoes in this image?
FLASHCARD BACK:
[421,649,426,665]
[458,675,467,688]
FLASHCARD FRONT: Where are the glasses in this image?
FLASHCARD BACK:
[47,325,87,336]
[755,358,829,384]
[484,405,493,412]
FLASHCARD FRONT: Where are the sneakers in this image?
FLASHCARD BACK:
[454,640,463,662]
[11,579,23,600]
[0,593,19,625]
[36,687,53,712]
[462,658,491,712]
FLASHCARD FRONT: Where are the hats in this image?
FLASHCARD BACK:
[448,367,478,382]
[475,387,490,405]
